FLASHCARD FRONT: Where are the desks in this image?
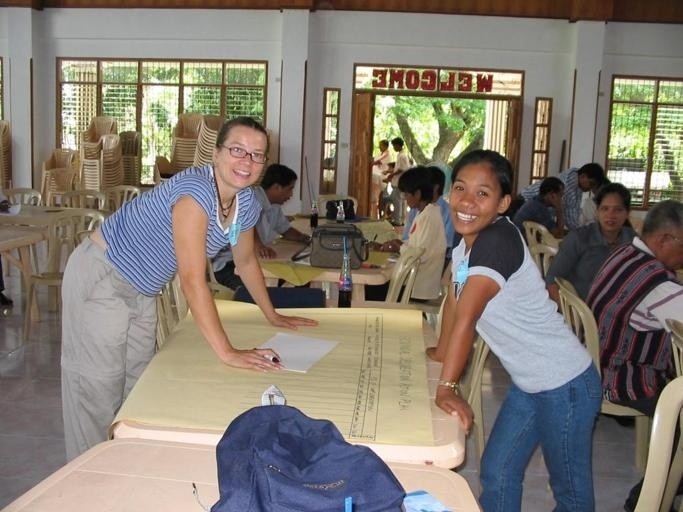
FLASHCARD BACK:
[234,218,403,308]
[115,316,466,470]
[1,203,97,312]
[0,438,483,511]
[1,226,43,323]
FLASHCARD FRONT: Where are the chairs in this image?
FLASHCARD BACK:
[23,207,106,341]
[155,284,176,348]
[0,120,12,186]
[459,336,491,458]
[634,376,683,512]
[521,220,558,247]
[547,275,652,479]
[386,246,426,303]
[40,115,143,191]
[60,188,107,230]
[529,243,557,275]
[205,257,235,294]
[2,188,42,277]
[105,185,142,215]
[153,111,268,184]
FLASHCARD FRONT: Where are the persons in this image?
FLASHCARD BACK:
[514,176,568,241]
[63,115,319,464]
[594,201,683,512]
[426,151,604,511]
[582,179,611,220]
[371,137,449,306]
[546,184,641,312]
[0,190,14,319]
[211,164,312,291]
[501,164,600,232]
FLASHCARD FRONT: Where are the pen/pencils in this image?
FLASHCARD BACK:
[373,234,377,243]
[46,210,63,212]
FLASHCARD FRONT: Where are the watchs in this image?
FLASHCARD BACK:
[438,379,461,395]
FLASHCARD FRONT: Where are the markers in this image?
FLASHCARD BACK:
[253,348,280,363]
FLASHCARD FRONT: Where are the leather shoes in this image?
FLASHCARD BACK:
[1,292,17,309]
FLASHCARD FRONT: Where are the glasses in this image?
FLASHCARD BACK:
[216,141,270,165]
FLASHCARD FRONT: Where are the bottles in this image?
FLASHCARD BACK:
[336,202,346,223]
[339,254,352,307]
[310,200,319,228]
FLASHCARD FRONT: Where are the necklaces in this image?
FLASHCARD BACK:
[212,163,236,218]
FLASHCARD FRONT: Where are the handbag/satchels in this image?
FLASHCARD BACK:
[289,221,372,270]
